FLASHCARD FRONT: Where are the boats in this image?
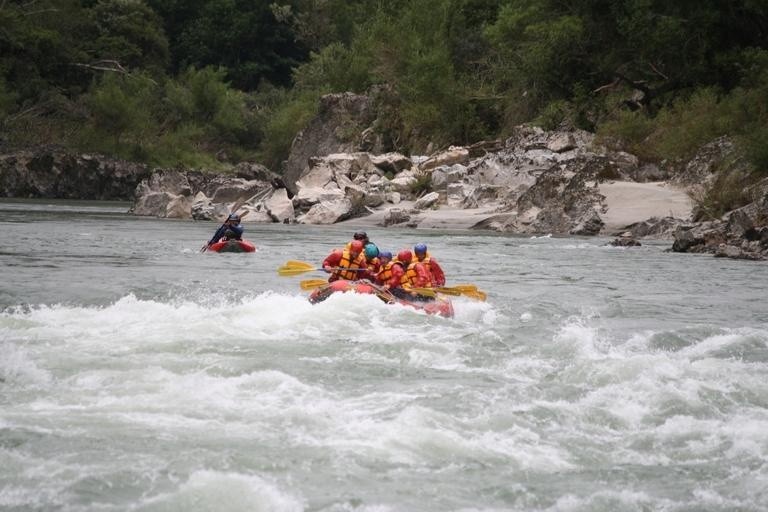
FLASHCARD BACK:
[208,238,256,252]
[309,279,454,319]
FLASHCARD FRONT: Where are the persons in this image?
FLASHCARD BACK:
[211,214,244,244]
[323,232,445,302]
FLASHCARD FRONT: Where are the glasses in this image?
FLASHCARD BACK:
[356,237,365,240]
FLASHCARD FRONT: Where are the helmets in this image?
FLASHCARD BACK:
[366,244,378,259]
[398,250,412,262]
[228,214,239,221]
[351,241,362,256]
[414,243,427,254]
[378,251,392,261]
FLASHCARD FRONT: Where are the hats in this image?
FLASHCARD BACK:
[354,232,366,238]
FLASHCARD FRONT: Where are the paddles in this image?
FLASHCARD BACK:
[200,198,249,255]
[408,284,486,302]
[278,261,368,291]
